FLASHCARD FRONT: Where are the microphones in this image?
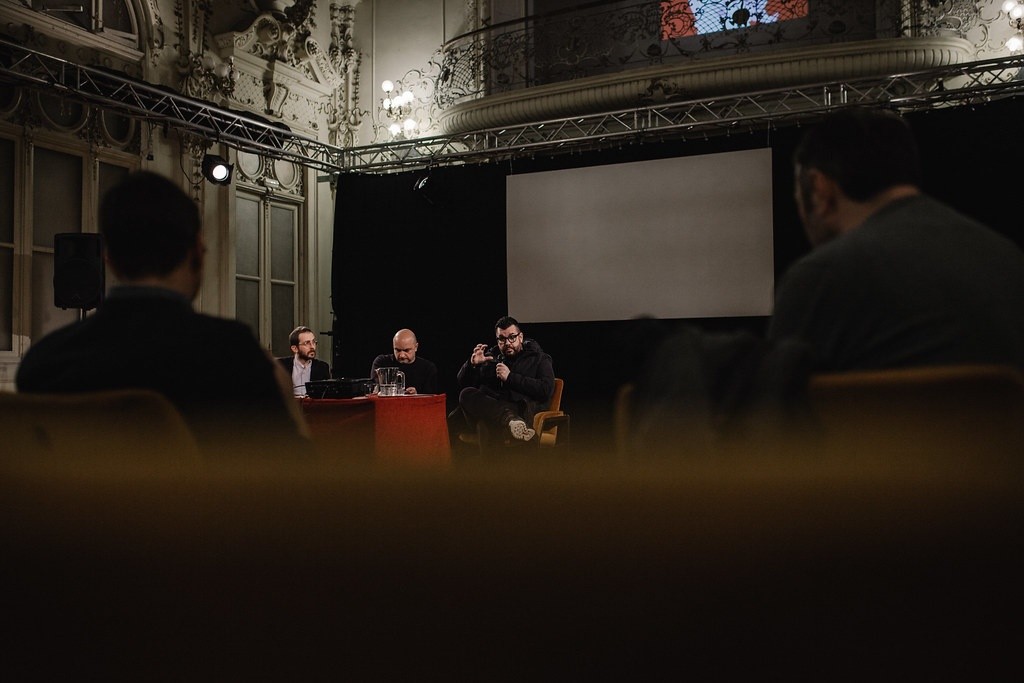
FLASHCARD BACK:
[496,354,504,388]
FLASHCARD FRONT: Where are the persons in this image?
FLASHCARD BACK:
[14,170,307,440]
[448,317,556,450]
[742,105,1024,422]
[275,326,331,398]
[371,329,439,394]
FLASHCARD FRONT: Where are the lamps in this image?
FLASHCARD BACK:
[412,176,445,207]
[1000,0,1024,55]
[382,79,414,120]
[200,153,234,186]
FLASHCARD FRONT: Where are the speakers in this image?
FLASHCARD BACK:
[52,232,105,311]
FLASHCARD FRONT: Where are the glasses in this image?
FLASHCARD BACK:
[298,339,319,346]
[497,331,521,344]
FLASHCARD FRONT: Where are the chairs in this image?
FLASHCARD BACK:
[456,378,570,456]
[808,365,1024,439]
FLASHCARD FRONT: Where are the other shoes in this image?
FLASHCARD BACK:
[505,415,536,442]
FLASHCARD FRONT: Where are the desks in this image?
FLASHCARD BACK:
[295,392,451,464]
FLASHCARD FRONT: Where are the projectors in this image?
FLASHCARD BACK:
[305,378,377,399]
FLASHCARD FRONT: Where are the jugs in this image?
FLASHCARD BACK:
[375,367,405,396]
